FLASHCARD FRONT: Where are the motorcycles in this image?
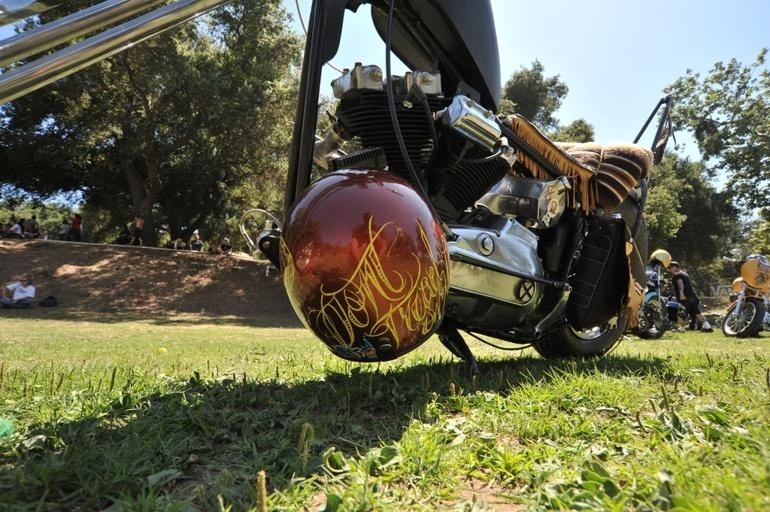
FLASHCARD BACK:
[622,249,684,340]
[0,0,681,375]
[715,254,770,337]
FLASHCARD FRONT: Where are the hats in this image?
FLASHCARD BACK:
[665,261,680,270]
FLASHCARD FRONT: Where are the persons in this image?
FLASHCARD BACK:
[0,272,35,308]
[348,211,444,267]
[669,260,711,333]
[666,296,684,329]
[0,212,233,256]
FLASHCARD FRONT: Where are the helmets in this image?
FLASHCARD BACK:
[731,275,745,293]
[276,165,453,366]
[739,258,770,290]
[648,247,673,271]
